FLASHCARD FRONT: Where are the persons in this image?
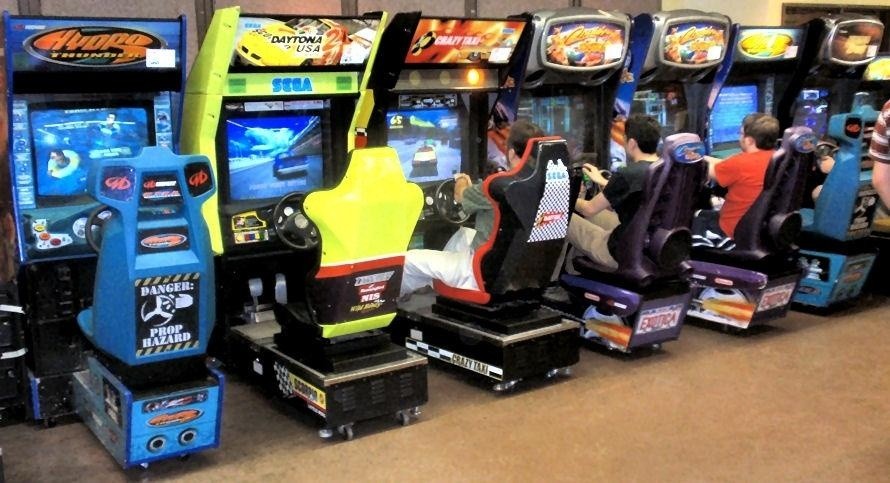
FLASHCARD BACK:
[692,111,782,250]
[550,113,665,294]
[395,119,546,297]
[867,97,889,209]
[105,113,117,127]
[49,149,69,164]
[811,155,835,203]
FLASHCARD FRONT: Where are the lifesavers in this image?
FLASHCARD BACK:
[48,150,79,178]
[97,123,120,135]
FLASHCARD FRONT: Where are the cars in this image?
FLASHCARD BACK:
[411,144,439,168]
[402,136,417,145]
[272,149,311,179]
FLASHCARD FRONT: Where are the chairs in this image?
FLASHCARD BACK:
[231,145,435,444]
[681,123,811,337]
[389,132,585,396]
[74,141,227,471]
[779,109,888,316]
[549,130,710,360]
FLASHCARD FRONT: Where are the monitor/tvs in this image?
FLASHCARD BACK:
[382,109,468,183]
[518,91,593,166]
[25,96,161,207]
[631,86,688,144]
[707,84,767,154]
[223,113,328,203]
[793,86,830,146]
